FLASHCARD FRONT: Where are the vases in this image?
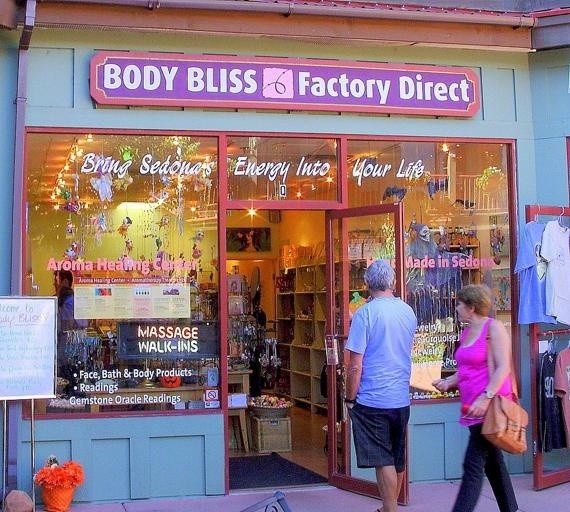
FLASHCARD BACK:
[39,484,77,512]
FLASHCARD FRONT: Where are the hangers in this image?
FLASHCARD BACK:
[558,202,568,227]
[534,200,543,222]
[545,326,555,352]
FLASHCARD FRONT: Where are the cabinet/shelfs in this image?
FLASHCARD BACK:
[272,256,372,419]
[435,244,480,304]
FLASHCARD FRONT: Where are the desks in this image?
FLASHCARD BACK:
[228,370,255,401]
[82,385,218,416]
[228,405,252,457]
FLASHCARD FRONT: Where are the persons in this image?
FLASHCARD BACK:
[431,284,521,512]
[344,258,418,511]
[54,270,89,399]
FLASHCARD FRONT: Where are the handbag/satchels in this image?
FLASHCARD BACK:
[482,391,530,453]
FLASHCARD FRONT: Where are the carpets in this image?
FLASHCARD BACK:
[226,452,335,494]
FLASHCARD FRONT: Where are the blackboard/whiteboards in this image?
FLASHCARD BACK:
[0,295,58,401]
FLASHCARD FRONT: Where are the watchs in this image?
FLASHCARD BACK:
[483,390,494,400]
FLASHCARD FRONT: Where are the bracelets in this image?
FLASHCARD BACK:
[344,398,357,405]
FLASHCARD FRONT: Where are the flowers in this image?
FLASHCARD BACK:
[31,454,87,492]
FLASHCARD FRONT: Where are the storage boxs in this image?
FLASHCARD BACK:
[249,414,292,455]
